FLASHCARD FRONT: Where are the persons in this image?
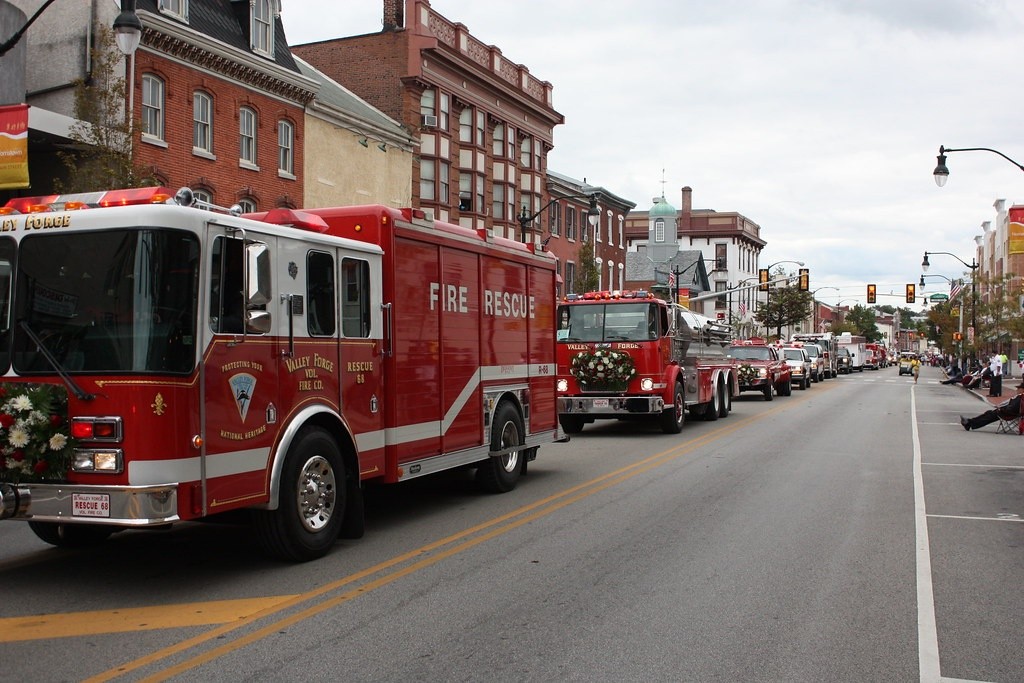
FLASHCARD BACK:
[910,356,921,384]
[960,383,1024,432]
[921,354,943,367]
[901,356,912,361]
[637,308,655,331]
[940,349,1008,397]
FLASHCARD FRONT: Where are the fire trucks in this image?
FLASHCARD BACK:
[555,288,741,435]
[0,184,571,566]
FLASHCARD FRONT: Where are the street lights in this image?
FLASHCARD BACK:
[673,257,729,315]
[516,192,600,243]
[919,274,964,371]
[766,261,805,337]
[836,298,859,336]
[921,251,980,374]
[804,286,840,335]
[728,278,777,342]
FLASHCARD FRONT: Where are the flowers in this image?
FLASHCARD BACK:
[737,364,758,386]
[0,385,72,483]
[569,343,635,390]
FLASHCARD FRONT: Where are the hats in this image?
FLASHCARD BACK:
[1015,383,1024,388]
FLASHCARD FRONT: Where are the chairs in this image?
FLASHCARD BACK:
[998,415,1023,435]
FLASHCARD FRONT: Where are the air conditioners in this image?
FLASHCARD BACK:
[421,115,437,127]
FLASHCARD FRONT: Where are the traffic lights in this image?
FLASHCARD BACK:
[906,284,915,303]
[953,331,964,341]
[909,332,912,339]
[920,332,924,340]
[895,332,900,339]
[867,284,876,303]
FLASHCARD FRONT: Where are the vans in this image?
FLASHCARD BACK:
[788,331,930,383]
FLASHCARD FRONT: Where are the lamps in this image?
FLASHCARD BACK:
[334,126,421,164]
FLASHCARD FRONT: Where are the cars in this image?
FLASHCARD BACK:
[837,347,855,374]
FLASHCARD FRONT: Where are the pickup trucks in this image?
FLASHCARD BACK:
[723,336,794,402]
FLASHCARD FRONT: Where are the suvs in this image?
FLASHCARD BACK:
[768,341,812,391]
[781,339,824,383]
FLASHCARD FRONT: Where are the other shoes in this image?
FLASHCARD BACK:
[914,381,916,383]
[960,415,970,431]
[989,395,998,397]
